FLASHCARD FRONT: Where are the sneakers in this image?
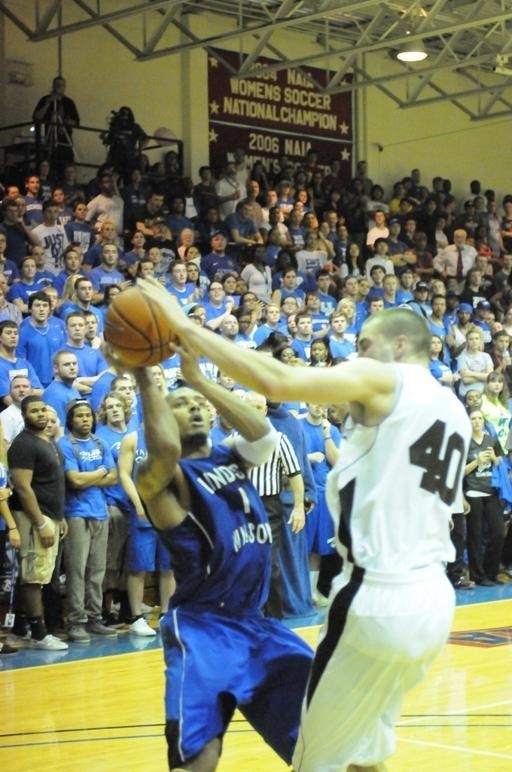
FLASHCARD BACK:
[5,629,32,643]
[449,574,475,589]
[111,599,152,616]
[128,616,156,636]
[28,633,68,651]
[101,611,126,628]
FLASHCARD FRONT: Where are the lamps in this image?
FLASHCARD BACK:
[398,11,430,65]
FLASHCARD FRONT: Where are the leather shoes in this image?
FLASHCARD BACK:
[67,622,90,643]
[470,576,496,587]
[0,644,18,653]
[85,620,117,637]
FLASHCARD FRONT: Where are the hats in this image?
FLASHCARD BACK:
[464,200,475,208]
[278,179,289,187]
[153,216,167,225]
[208,230,225,238]
[65,397,90,413]
[388,217,399,225]
[42,200,56,210]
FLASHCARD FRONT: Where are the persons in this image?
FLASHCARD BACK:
[24,200,69,278]
[63,200,95,270]
[85,162,115,204]
[57,278,104,351]
[297,401,343,603]
[54,247,88,305]
[0,376,66,638]
[59,163,87,205]
[32,76,80,187]
[450,173,512,588]
[83,173,125,248]
[66,241,88,289]
[41,352,83,436]
[57,399,117,643]
[1,199,41,268]
[0,273,23,328]
[7,395,68,651]
[119,416,177,636]
[1,420,21,654]
[222,392,306,625]
[88,222,128,281]
[136,273,472,772]
[36,159,53,213]
[120,149,359,273]
[0,185,19,209]
[136,272,360,363]
[1,231,21,298]
[0,319,45,413]
[51,185,73,227]
[95,392,138,626]
[68,242,85,268]
[15,197,29,235]
[100,332,318,772]
[47,311,107,405]
[8,256,59,320]
[31,246,57,287]
[83,312,103,351]
[14,291,68,390]
[13,245,56,289]
[100,106,149,175]
[359,147,449,389]
[23,176,44,231]
[90,243,132,329]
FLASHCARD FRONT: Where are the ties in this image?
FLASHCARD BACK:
[456,248,463,283]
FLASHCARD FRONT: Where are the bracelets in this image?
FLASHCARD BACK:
[37,521,49,529]
[8,527,17,532]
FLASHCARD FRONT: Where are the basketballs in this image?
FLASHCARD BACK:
[107,285,181,367]
